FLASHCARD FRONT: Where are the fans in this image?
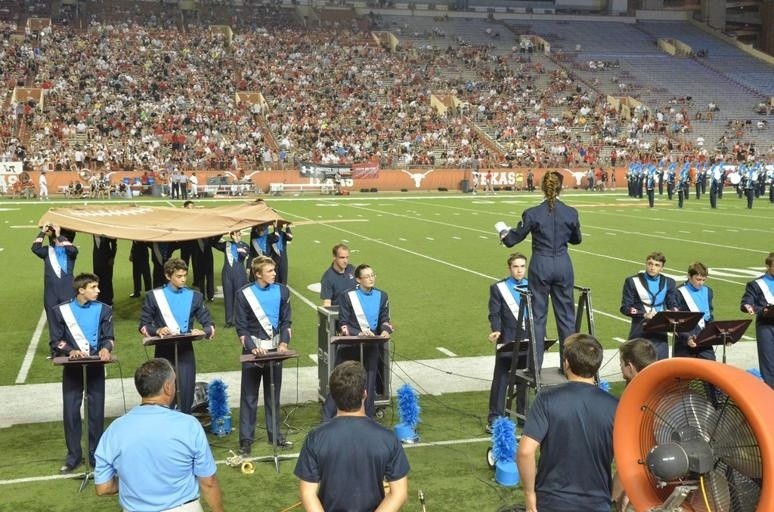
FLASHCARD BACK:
[612,356,774,512]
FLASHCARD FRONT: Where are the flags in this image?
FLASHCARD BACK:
[38,201,294,240]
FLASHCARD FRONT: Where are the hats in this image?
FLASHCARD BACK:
[205,381,231,433]
[394,384,420,445]
[493,418,519,487]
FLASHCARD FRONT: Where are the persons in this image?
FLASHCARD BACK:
[235,254,292,455]
[672,262,720,409]
[515,333,627,512]
[173,200,193,269]
[319,245,361,307]
[264,221,292,289]
[695,160,701,199]
[619,252,678,359]
[320,265,392,429]
[187,238,215,299]
[733,162,774,209]
[93,236,117,309]
[700,159,709,194]
[626,82,773,163]
[677,161,683,208]
[684,168,690,200]
[494,170,583,378]
[93,359,225,512]
[666,162,676,200]
[48,225,76,248]
[740,253,773,389]
[294,360,410,512]
[710,162,720,208]
[49,272,114,474]
[718,165,723,199]
[247,221,276,283]
[625,162,655,208]
[148,240,175,290]
[212,229,249,330]
[662,47,709,60]
[656,159,667,195]
[129,238,153,299]
[31,224,78,360]
[139,257,216,414]
[1,0,625,200]
[619,338,659,383]
[487,252,546,432]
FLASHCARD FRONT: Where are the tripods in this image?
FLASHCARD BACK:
[722,343,727,363]
[53,366,119,494]
[174,343,179,414]
[239,364,300,473]
[672,330,675,358]
[525,354,529,420]
[360,344,365,367]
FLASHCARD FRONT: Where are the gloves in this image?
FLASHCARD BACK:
[494,222,511,233]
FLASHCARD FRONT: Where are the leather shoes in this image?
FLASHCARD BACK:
[46,353,59,360]
[209,297,214,302]
[224,322,232,328]
[268,438,293,450]
[129,293,141,299]
[486,426,493,434]
[558,368,566,375]
[60,464,74,474]
[237,441,251,457]
[523,369,540,380]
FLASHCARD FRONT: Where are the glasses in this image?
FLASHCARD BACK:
[360,272,375,278]
